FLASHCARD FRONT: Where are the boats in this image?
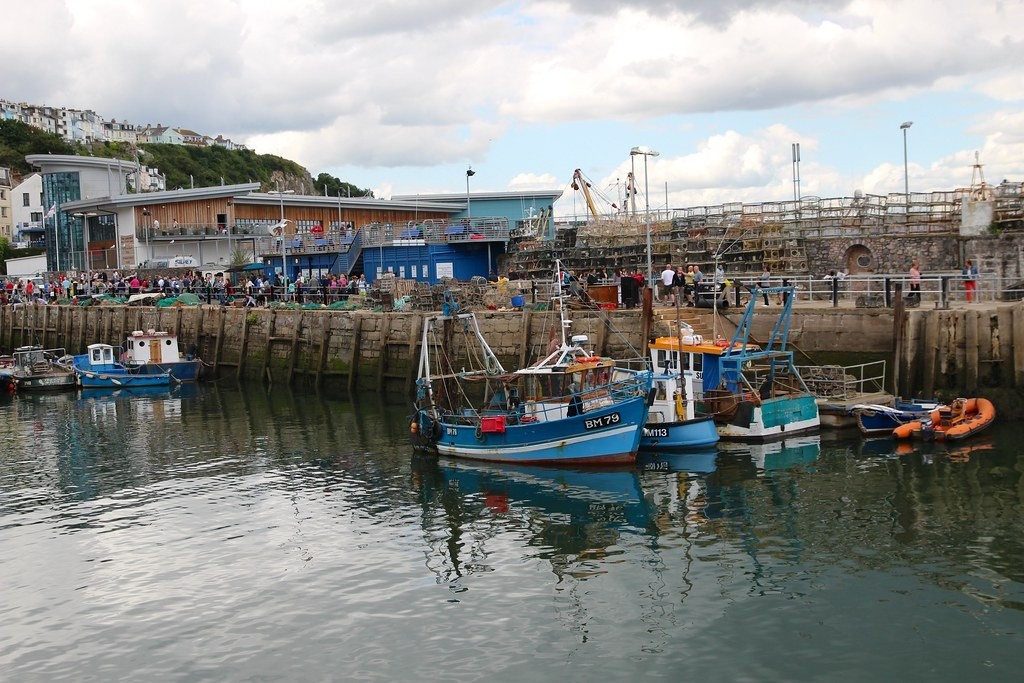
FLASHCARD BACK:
[718,441,822,600]
[0,374,14,403]
[647,286,822,444]
[643,446,718,528]
[118,327,204,383]
[124,378,198,429]
[894,443,996,466]
[0,355,15,375]
[484,260,721,452]
[893,397,997,441]
[406,453,658,571]
[70,343,173,387]
[851,401,947,434]
[11,343,78,393]
[855,438,900,470]
[408,291,650,466]
[74,386,122,428]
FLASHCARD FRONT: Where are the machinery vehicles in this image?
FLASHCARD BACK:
[506,205,553,254]
[571,168,601,225]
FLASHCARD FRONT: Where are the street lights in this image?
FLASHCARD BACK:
[467,170,476,219]
[338,187,344,232]
[630,148,659,292]
[415,193,420,220]
[900,122,914,232]
[141,208,153,260]
[74,213,97,269]
[268,190,295,301]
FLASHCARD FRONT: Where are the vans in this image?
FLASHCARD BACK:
[18,277,51,288]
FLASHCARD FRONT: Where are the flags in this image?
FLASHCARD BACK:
[44,205,55,219]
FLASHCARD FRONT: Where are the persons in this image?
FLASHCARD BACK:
[308,274,318,304]
[294,227,302,253]
[671,266,686,303]
[599,265,609,285]
[80,269,153,299]
[677,266,695,284]
[153,217,160,236]
[756,265,770,306]
[288,273,367,306]
[310,222,323,251]
[960,260,978,303]
[693,266,703,292]
[508,269,519,281]
[271,227,283,253]
[560,271,590,301]
[714,265,726,291]
[907,260,922,301]
[340,223,353,249]
[488,273,507,292]
[238,272,290,308]
[651,266,662,303]
[612,267,645,309]
[587,268,599,286]
[0,273,81,305]
[172,219,181,236]
[488,270,498,282]
[152,269,234,305]
[661,264,675,306]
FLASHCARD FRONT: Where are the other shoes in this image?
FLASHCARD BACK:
[663,303,667,306]
[686,301,694,307]
[671,302,675,306]
